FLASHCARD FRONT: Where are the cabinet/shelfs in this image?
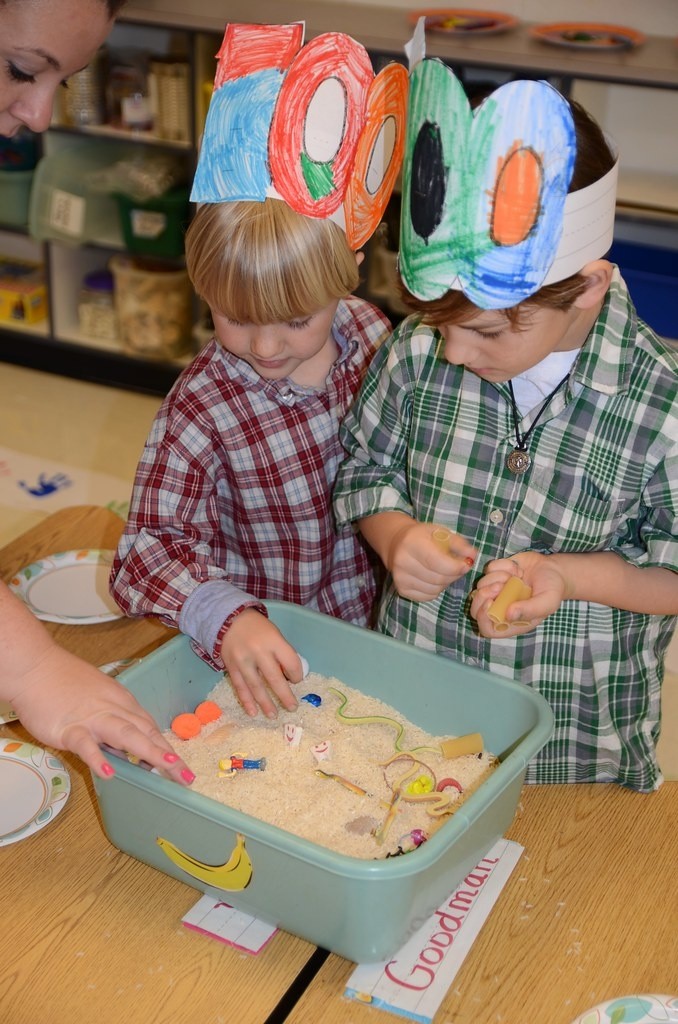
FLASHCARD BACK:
[0,0,678,398]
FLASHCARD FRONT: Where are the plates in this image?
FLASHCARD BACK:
[8,550,137,626]
[528,24,647,52]
[0,737,71,848]
[0,701,20,725]
[569,994,678,1024]
[408,9,517,37]
[97,658,139,678]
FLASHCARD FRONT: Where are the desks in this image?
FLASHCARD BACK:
[0,504,678,1024]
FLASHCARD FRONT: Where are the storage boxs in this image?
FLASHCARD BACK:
[87,593,555,965]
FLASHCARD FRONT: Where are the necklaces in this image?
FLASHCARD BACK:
[507,374,570,474]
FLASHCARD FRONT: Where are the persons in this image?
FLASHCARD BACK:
[0,0,197,787]
[327,17,678,794]
[108,20,408,720]
